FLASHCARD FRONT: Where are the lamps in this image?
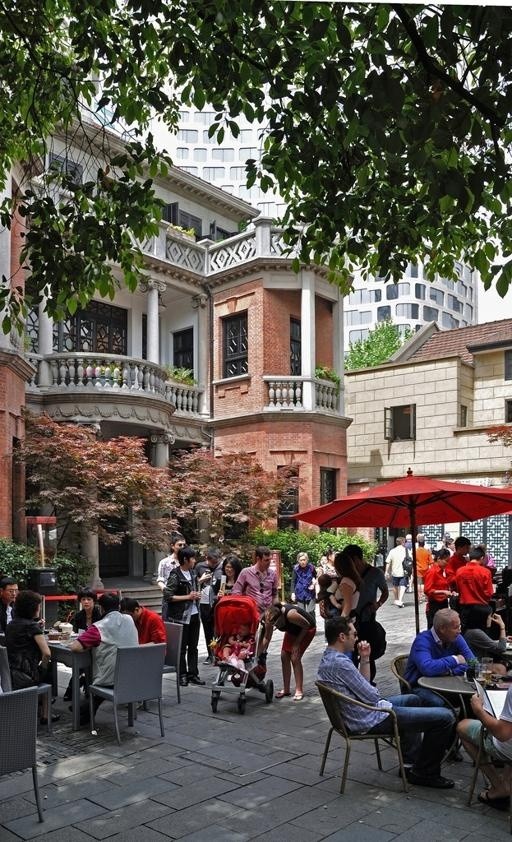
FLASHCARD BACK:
[158,291,167,313]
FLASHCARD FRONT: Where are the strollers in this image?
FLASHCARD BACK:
[209,594,275,717]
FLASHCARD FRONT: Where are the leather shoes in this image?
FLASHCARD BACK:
[38,691,78,724]
[178,674,205,687]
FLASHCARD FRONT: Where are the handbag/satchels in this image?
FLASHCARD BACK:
[402,557,413,577]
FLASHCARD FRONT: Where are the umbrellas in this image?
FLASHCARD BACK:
[289,468,512,634]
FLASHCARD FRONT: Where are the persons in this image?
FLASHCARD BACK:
[233,547,280,692]
[214,534,240,681]
[405,609,479,761]
[385,535,413,606]
[458,683,512,809]
[194,545,223,666]
[156,531,186,622]
[264,601,316,700]
[120,597,168,656]
[318,620,456,787]
[0,577,46,634]
[164,546,205,686]
[416,535,512,654]
[293,544,389,686]
[63,587,104,702]
[68,593,141,726]
[223,622,255,670]
[0,591,61,726]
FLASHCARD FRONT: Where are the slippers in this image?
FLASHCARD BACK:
[276,690,291,698]
[293,693,303,701]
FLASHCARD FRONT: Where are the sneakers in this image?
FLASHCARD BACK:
[445,746,463,764]
[399,764,455,788]
[393,600,403,607]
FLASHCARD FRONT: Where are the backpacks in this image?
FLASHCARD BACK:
[354,620,386,659]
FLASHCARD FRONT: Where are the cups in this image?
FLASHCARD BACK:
[48,622,80,647]
[463,655,494,691]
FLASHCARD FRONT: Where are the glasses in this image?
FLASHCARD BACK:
[339,632,357,636]
[451,623,460,629]
[260,583,264,593]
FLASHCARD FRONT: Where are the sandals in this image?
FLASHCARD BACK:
[478,791,508,803]
